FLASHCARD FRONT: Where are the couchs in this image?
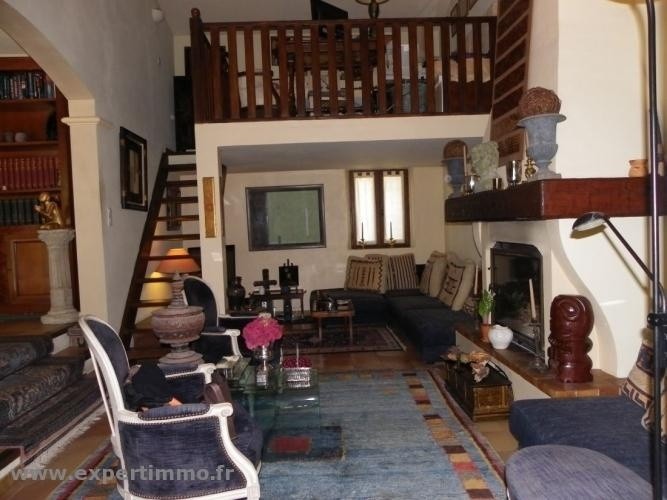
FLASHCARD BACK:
[311,286,474,365]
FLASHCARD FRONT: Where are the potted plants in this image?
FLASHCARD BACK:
[474,289,500,346]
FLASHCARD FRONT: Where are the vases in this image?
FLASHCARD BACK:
[440,158,468,200]
[516,113,569,184]
[254,344,274,385]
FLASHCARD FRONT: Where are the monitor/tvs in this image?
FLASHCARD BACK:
[489,241,546,359]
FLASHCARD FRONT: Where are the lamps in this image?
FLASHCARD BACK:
[572,210,667,327]
[354,0,392,39]
[155,248,204,311]
[150,8,166,24]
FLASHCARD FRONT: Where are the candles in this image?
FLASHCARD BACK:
[463,146,469,178]
[524,129,532,152]
[390,221,394,239]
[361,224,365,239]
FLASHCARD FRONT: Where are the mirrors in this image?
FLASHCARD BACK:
[244,184,329,253]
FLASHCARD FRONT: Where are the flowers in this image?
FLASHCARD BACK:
[242,316,285,350]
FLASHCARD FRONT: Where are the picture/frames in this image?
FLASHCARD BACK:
[448,0,481,39]
[117,124,150,214]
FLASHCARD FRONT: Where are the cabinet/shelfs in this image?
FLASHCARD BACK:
[441,358,516,426]
[173,73,197,154]
[0,55,79,318]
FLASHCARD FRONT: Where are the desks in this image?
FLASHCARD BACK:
[225,307,267,316]
[272,34,394,120]
[249,288,309,322]
[503,440,655,500]
[221,366,322,465]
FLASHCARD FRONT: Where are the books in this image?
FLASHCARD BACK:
[0,156,63,190]
[1,70,56,98]
[0,198,40,225]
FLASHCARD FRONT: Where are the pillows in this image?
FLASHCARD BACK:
[439,254,477,314]
[641,371,667,449]
[345,255,389,297]
[386,254,421,292]
[418,250,449,300]
[613,324,667,413]
[365,254,390,266]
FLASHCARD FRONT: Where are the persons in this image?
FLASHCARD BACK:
[32,192,65,229]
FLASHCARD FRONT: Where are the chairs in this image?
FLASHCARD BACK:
[505,314,667,500]
[220,47,288,121]
[77,309,263,500]
[181,274,259,363]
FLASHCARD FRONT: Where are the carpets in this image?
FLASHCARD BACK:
[1,333,111,482]
[44,362,512,500]
[281,323,410,359]
